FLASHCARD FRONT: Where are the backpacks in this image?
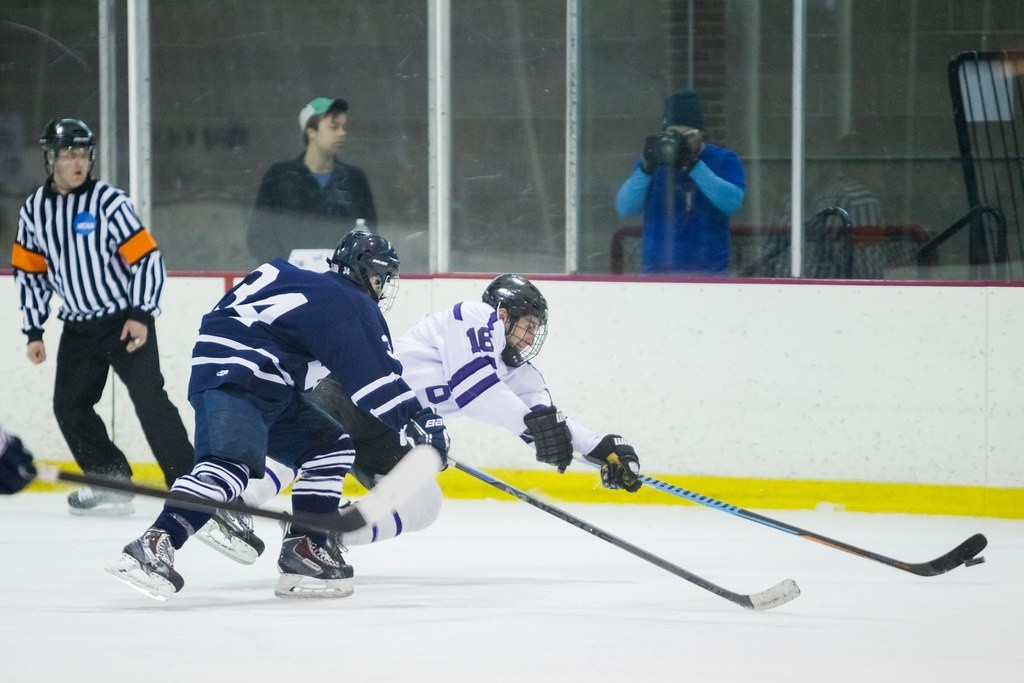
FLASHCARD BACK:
[584,433,641,493]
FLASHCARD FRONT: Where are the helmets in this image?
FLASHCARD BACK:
[331,231,401,297]
[40,116,97,166]
[482,272,548,366]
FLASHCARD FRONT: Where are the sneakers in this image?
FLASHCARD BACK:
[269,524,355,598]
[188,507,264,564]
[66,480,139,514]
[106,527,185,602]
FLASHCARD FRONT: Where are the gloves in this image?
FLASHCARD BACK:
[523,406,573,474]
[671,131,696,179]
[399,406,451,474]
[639,132,663,176]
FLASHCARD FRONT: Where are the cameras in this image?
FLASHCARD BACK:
[654,130,691,163]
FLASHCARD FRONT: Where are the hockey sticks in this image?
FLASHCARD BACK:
[27,443,442,532]
[635,473,986,578]
[445,457,800,612]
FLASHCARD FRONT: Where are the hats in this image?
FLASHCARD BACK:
[299,97,349,135]
[662,89,704,132]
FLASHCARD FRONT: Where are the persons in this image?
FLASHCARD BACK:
[614,90,746,277]
[12,118,194,520]
[764,134,884,280]
[106,230,450,603]
[246,98,377,268]
[193,273,643,568]
